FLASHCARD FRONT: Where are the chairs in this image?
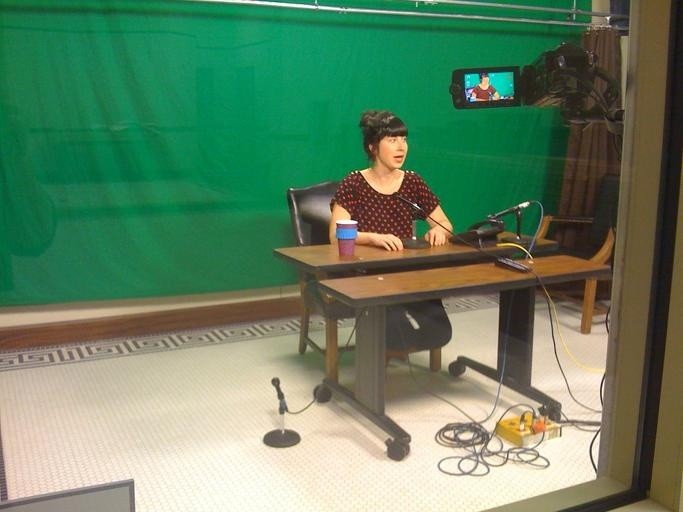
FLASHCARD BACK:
[537,174,619,334]
[286,181,355,357]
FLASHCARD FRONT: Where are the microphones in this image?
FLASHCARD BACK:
[271,377,288,412]
[392,192,532,272]
[487,202,530,221]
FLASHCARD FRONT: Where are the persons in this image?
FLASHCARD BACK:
[469,72,499,102]
[327,109,456,366]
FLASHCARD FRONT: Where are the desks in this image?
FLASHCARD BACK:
[275,228,614,461]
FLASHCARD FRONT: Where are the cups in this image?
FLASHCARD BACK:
[336,220,358,259]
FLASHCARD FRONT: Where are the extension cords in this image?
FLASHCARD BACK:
[496,411,562,448]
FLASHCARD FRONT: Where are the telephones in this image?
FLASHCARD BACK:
[448,219,505,247]
